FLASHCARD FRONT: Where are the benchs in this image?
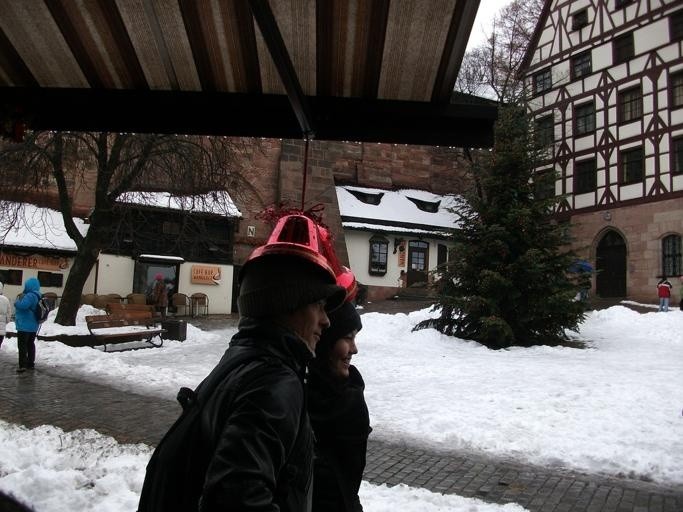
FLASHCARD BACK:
[85,315,169,352]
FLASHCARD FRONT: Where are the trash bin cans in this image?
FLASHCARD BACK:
[161,319,187,342]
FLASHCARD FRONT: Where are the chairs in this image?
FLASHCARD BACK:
[17,292,209,318]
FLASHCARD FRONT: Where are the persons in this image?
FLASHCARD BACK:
[152,273,167,321]
[167,281,176,312]
[13,277,42,372]
[0,273,11,347]
[137,252,347,512]
[578,270,591,301]
[656,272,683,311]
[305,301,373,512]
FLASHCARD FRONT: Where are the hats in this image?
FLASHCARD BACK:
[235,254,347,319]
[315,300,361,357]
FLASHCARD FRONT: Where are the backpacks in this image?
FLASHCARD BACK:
[137,348,309,512]
[29,291,50,323]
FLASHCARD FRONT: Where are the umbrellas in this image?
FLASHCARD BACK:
[566,258,592,273]
